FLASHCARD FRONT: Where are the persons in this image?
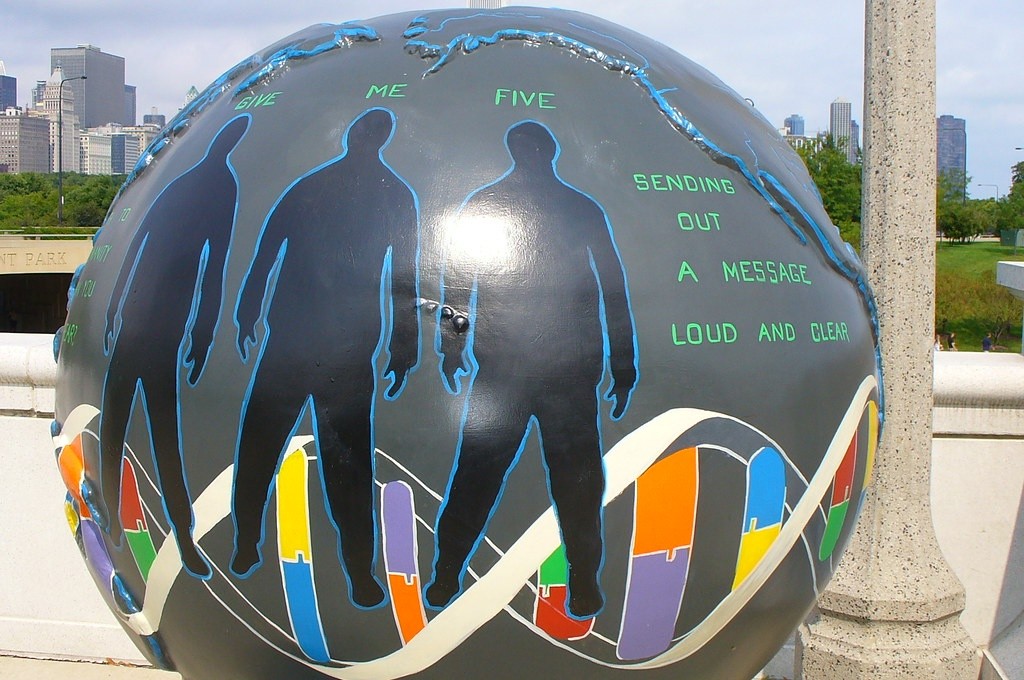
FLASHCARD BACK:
[935,331,958,351]
[982,333,997,352]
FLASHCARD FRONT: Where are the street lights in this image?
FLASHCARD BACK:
[942,127,967,206]
[978,183,999,202]
[57,76,88,225]
